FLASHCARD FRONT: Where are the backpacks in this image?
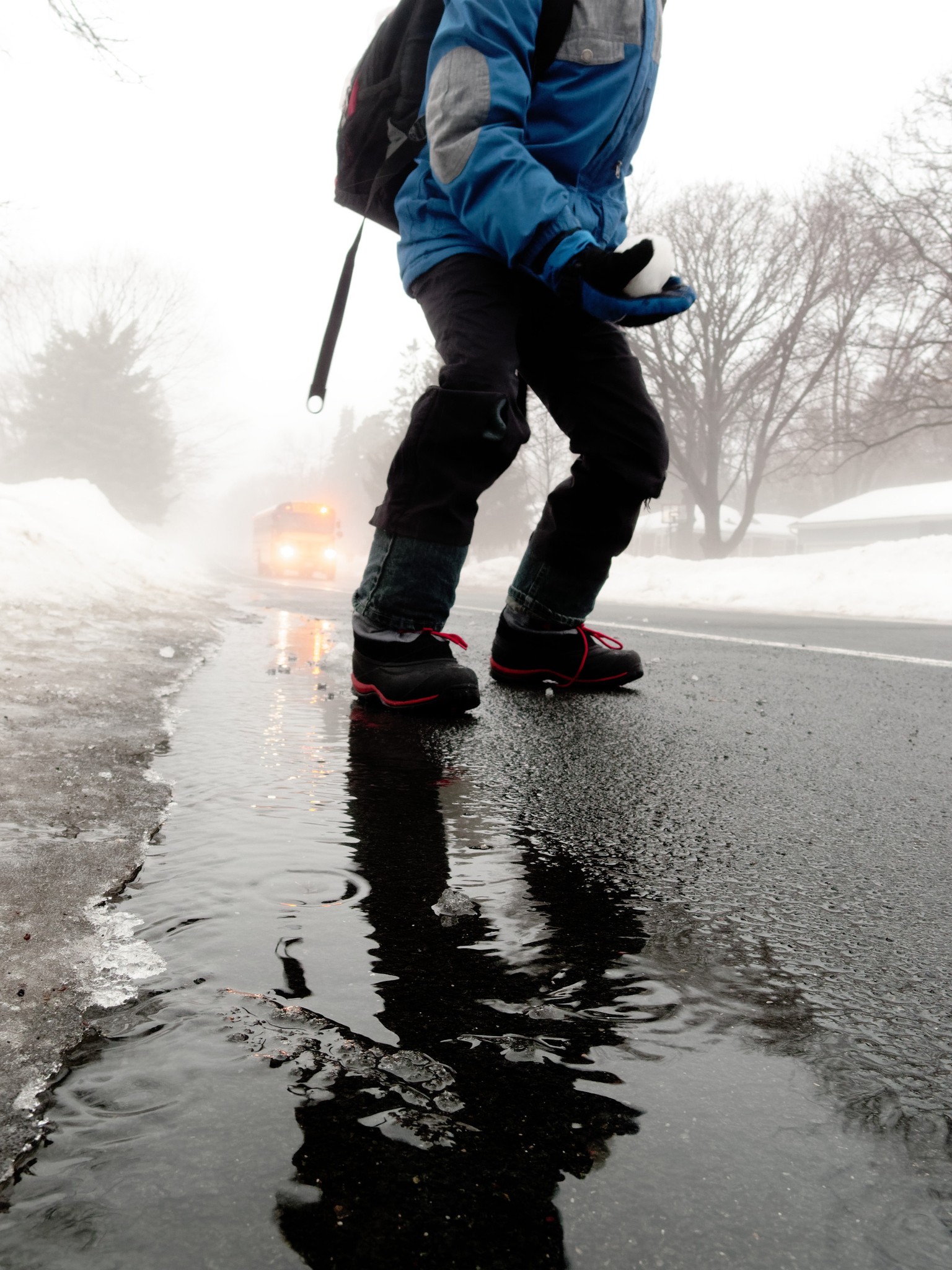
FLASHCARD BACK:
[334,0,573,239]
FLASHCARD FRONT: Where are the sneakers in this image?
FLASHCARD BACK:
[351,614,480,713]
[488,609,643,688]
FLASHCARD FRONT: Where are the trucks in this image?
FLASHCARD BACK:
[252,499,343,581]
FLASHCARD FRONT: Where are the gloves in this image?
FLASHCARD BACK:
[558,237,696,327]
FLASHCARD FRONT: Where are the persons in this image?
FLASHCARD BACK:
[352,0,696,711]
